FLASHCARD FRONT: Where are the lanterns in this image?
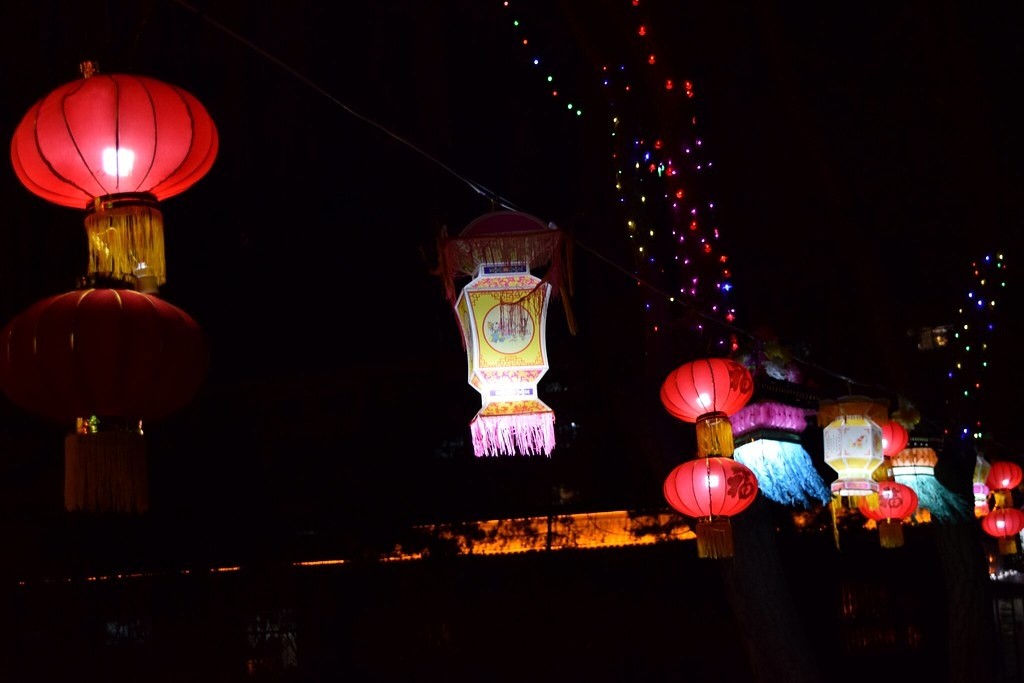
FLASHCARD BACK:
[2,271,205,516]
[650,343,1024,569]
[443,205,576,462]
[7,57,220,293]
